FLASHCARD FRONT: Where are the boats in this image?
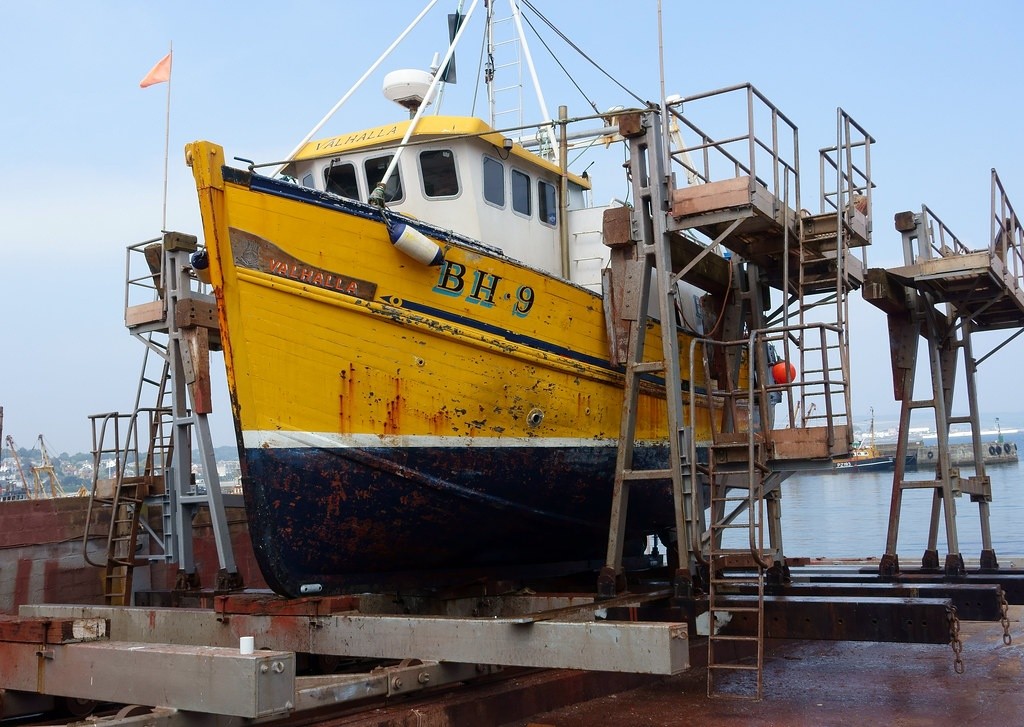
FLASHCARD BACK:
[185,0,797,599]
[829,408,894,470]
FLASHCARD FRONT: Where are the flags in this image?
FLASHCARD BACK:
[140,53,171,90]
